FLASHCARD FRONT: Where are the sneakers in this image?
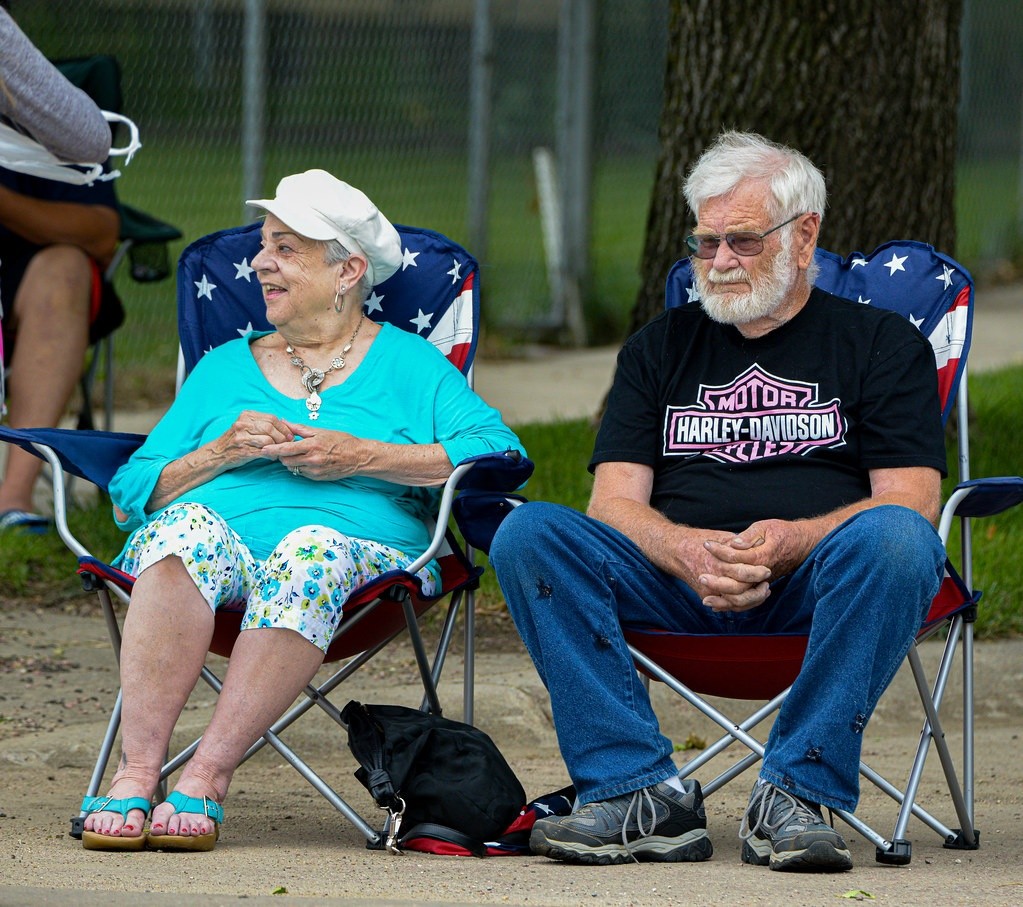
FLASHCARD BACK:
[739,774,853,872]
[529,778,713,864]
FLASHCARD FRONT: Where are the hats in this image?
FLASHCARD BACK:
[243,168,404,286]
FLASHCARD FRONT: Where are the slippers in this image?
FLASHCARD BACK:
[81,795,150,851]
[146,791,225,851]
[0,511,50,534]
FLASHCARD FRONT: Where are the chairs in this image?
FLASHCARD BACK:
[0,223,534,849]
[453,244,1023,864]
[0,59,181,513]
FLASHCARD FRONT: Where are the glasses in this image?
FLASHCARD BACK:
[684,212,818,260]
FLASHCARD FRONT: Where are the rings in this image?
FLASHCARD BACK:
[293,466,302,477]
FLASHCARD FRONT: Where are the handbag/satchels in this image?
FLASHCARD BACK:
[0,169,120,267]
[339,699,528,836]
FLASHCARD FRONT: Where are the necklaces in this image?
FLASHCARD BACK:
[283,313,368,422]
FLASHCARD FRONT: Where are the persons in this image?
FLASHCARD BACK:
[0,9,120,530]
[78,169,535,853]
[489,121,947,875]
